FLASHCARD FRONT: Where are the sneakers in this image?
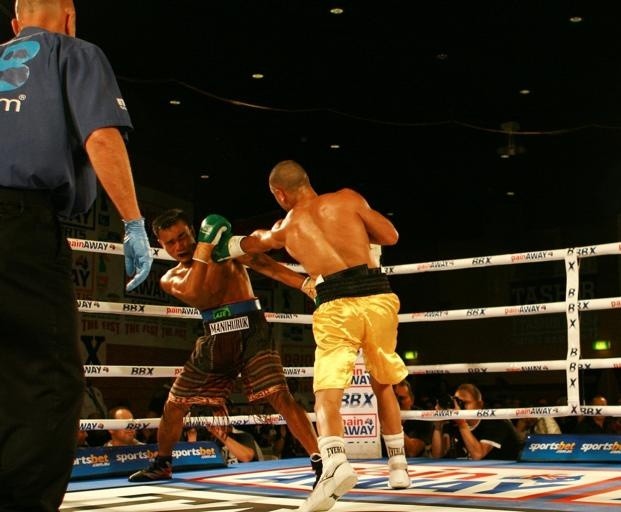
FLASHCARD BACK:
[298,453,358,512]
[128,456,172,481]
[389,455,410,488]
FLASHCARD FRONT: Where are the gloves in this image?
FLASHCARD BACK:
[123,218,153,291]
[192,214,247,264]
[301,277,320,308]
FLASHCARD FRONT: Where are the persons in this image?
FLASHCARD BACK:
[105,408,148,446]
[76,430,89,447]
[0,1,152,511]
[391,379,426,457]
[431,382,621,460]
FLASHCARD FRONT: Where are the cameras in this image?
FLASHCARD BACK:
[438,393,465,410]
